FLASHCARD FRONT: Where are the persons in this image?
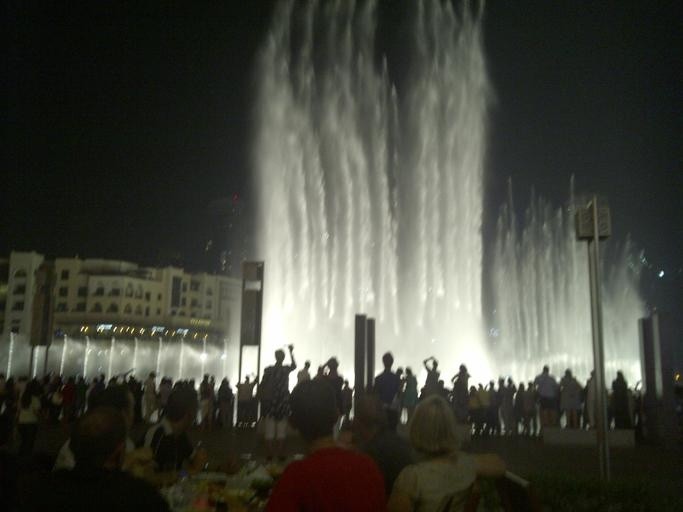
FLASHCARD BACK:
[374,351,400,419]
[260,346,297,460]
[2,357,682,512]
